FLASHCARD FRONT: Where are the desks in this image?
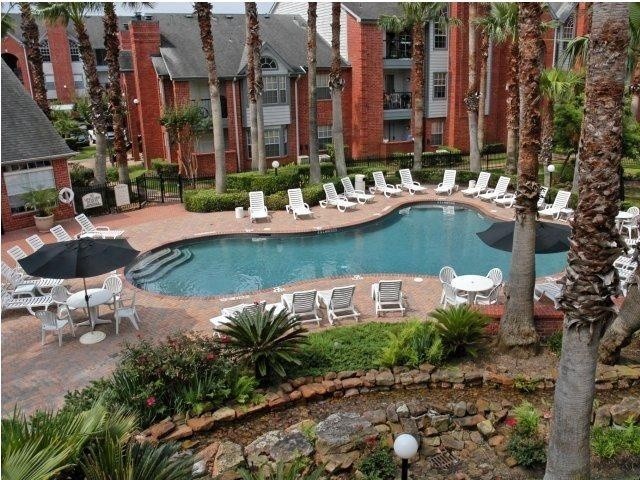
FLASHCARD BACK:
[469,180,476,189]
[559,208,574,223]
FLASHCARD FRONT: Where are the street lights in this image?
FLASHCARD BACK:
[547,163,557,203]
[392,430,422,480]
[271,158,280,176]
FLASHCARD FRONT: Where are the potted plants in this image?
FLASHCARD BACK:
[19,183,64,235]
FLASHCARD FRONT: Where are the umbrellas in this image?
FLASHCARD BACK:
[473,218,572,255]
[16,235,142,336]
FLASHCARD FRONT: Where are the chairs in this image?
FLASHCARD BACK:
[439,265,568,311]
[395,169,428,195]
[611,206,640,294]
[434,170,460,196]
[285,188,314,220]
[537,186,549,210]
[209,283,363,336]
[461,171,491,199]
[1,212,140,348]
[538,190,571,220]
[340,176,376,205]
[478,175,511,203]
[372,279,408,317]
[319,182,358,213]
[369,171,402,198]
[248,190,268,222]
[493,191,517,208]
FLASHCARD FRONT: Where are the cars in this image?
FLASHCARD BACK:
[71,132,90,146]
[93,125,115,144]
[75,117,88,130]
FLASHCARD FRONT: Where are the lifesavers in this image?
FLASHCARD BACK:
[58,189,75,203]
